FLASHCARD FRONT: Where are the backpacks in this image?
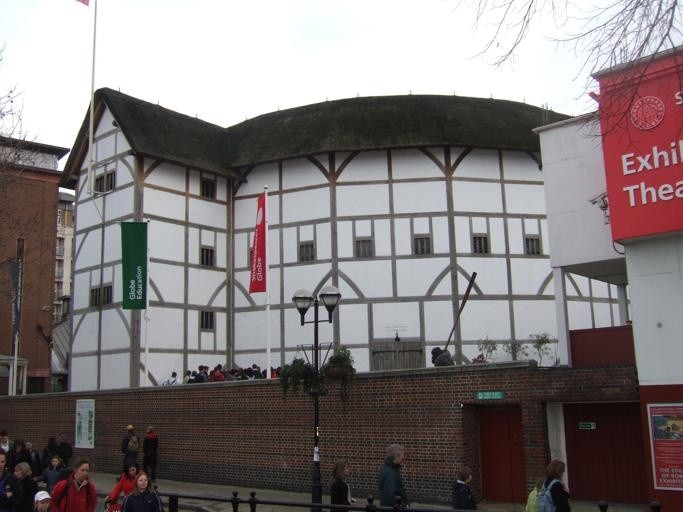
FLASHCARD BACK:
[126,436,138,453]
[536,480,562,512]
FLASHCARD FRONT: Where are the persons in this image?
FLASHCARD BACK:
[161,364,282,387]
[543,459,571,512]
[58,379,66,391]
[431,346,455,368]
[525,478,544,512]
[379,444,411,508]
[330,461,357,512]
[452,465,477,510]
[0,424,165,512]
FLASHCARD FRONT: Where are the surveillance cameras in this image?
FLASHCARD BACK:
[587,189,608,205]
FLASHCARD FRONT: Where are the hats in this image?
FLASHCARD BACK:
[34,491,50,504]
[126,424,135,430]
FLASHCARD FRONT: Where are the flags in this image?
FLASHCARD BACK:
[120,222,147,310]
[248,191,265,294]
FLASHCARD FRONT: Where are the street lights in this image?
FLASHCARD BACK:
[292,286,341,511]
